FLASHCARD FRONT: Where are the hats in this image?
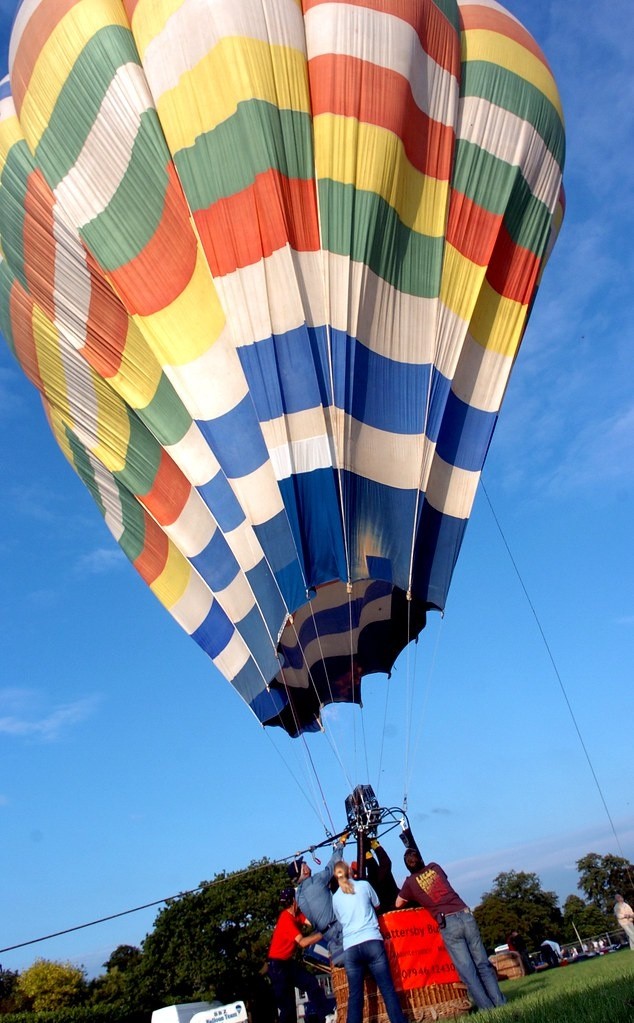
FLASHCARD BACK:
[287,855,303,883]
[279,887,299,909]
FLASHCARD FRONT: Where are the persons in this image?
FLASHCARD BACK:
[561,939,607,957]
[395,848,508,1011]
[613,894,634,951]
[365,840,404,917]
[331,861,405,1023]
[288,833,347,956]
[266,889,334,1023]
[507,930,535,975]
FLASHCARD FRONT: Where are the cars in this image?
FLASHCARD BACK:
[523,938,629,973]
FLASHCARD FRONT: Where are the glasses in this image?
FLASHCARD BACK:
[300,862,306,867]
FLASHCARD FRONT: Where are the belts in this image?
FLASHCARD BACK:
[319,920,339,935]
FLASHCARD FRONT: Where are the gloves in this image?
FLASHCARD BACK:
[399,819,407,830]
[340,831,350,847]
[365,851,372,860]
[370,837,380,850]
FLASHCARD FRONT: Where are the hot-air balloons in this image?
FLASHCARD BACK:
[1,1,565,1023]
